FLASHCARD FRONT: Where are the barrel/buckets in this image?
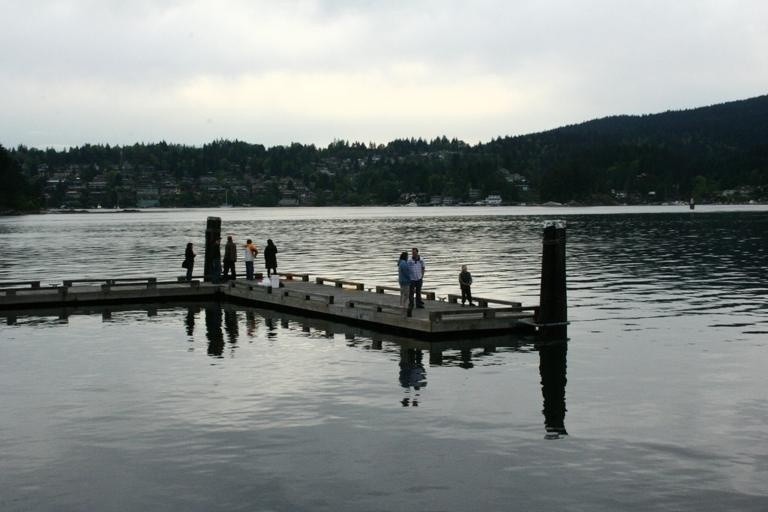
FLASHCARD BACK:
[271,275,279,288]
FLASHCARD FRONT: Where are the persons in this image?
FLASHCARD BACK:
[459,265,475,307]
[244,240,258,280]
[223,236,237,274]
[264,239,278,275]
[397,252,410,306]
[182,243,196,278]
[408,248,424,308]
